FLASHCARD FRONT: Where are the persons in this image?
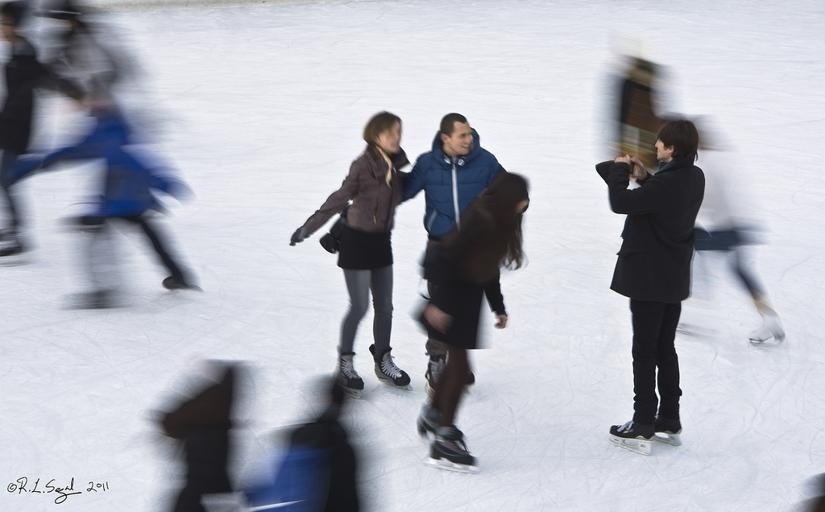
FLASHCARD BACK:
[594,119,704,441]
[619,29,793,342]
[1,0,202,309]
[235,380,360,512]
[409,172,530,465]
[291,112,411,389]
[403,112,506,389]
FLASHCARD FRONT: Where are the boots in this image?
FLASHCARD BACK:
[419,403,476,468]
[654,410,684,435]
[745,316,783,341]
[608,419,655,443]
[366,343,411,388]
[335,347,365,390]
